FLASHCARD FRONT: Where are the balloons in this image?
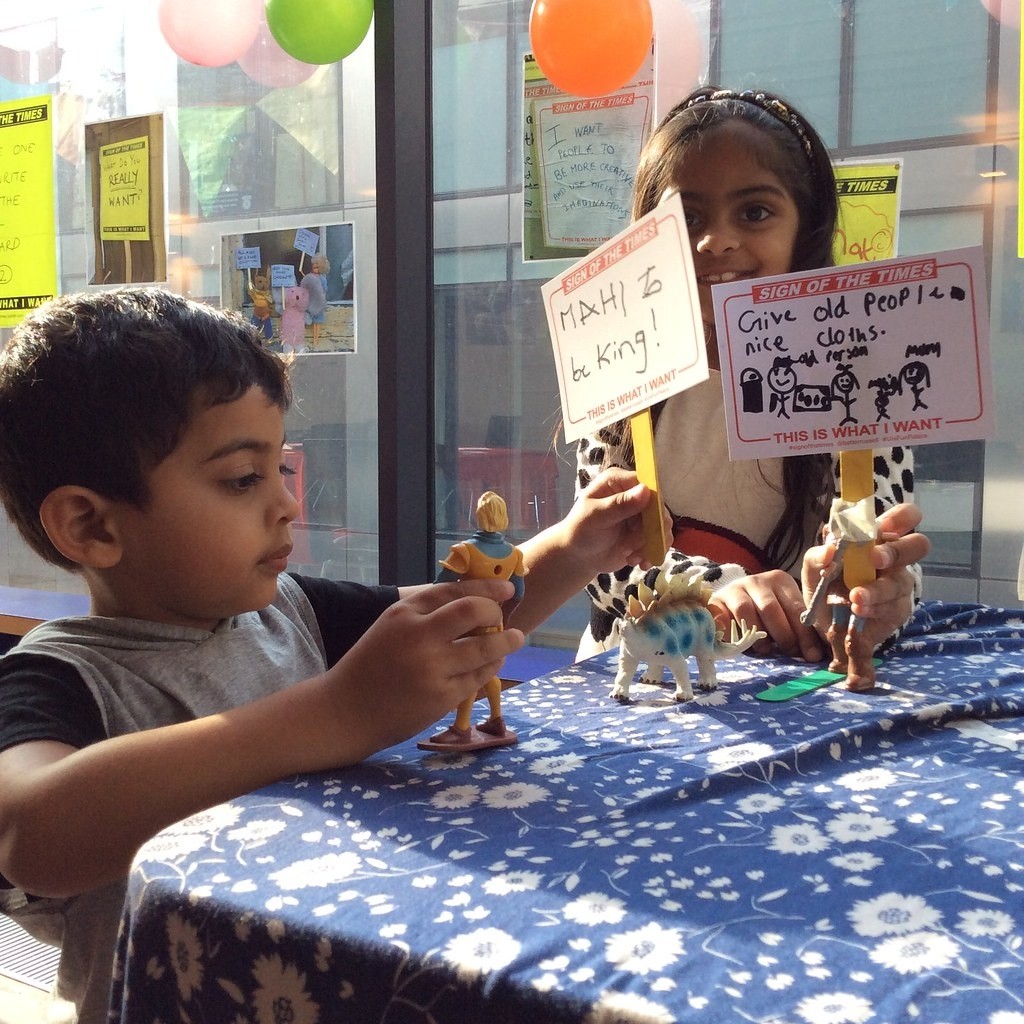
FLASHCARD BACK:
[528,0,652,98]
[237,0,321,87]
[265,0,373,65]
[158,0,263,67]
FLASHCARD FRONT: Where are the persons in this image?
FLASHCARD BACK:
[300,253,330,345]
[576,86,927,670]
[428,491,530,743]
[0,287,674,917]
[801,501,899,690]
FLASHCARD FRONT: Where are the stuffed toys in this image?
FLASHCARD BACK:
[247,276,274,347]
[275,287,312,355]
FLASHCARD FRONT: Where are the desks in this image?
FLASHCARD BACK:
[104,595,1024,1024]
